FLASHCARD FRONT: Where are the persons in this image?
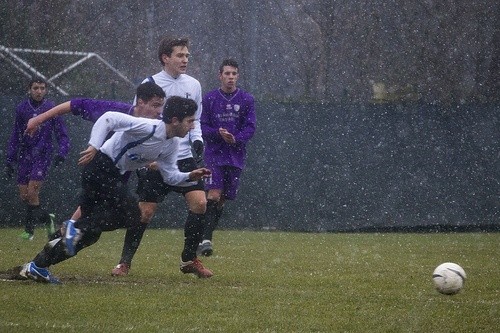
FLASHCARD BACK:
[113,33,213,279]
[5,78,72,240]
[24,96,211,283]
[12,81,166,278]
[198,59,256,255]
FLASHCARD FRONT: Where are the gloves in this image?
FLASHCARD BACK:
[194,140,204,163]
[3,167,12,178]
[54,155,63,168]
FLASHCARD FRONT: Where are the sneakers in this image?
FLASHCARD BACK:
[180,259,213,278]
[18,230,34,240]
[19,262,61,284]
[61,220,84,256]
[45,214,56,238]
[202,241,213,256]
[110,262,128,276]
[197,245,202,257]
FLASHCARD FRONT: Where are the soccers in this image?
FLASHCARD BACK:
[432,262,467,296]
